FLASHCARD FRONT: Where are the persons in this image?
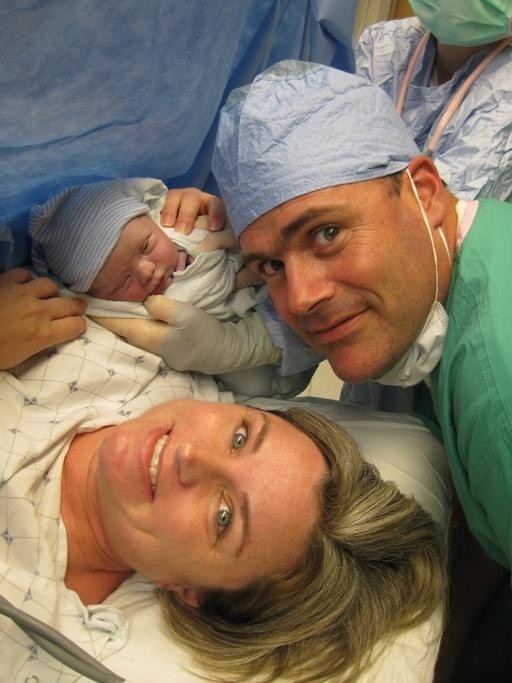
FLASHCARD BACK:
[1,267,458,683]
[354,0,509,200]
[162,58,510,578]
[26,173,320,399]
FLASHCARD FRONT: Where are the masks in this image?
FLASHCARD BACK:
[374,173,452,386]
[407,0,512,46]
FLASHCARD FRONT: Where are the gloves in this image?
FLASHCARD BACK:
[85,294,281,376]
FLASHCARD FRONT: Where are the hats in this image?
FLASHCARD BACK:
[28,186,148,293]
[212,60,419,239]
